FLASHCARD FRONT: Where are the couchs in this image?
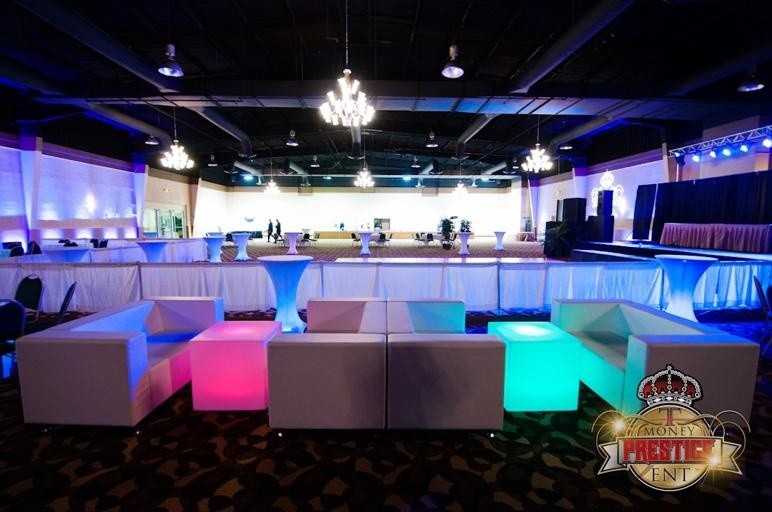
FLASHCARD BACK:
[550,300,760,427]
[387,300,506,432]
[267,297,387,428]
[15,296,224,428]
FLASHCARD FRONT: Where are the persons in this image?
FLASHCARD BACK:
[267,219,275,242]
[275,219,282,240]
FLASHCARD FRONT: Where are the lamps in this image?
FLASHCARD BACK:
[318,0,376,128]
[442,3,464,79]
[157,0,185,79]
[674,129,772,167]
[521,116,553,175]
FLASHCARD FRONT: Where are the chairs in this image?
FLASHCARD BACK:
[9,239,109,258]
[0,274,109,378]
[352,232,393,248]
[412,232,457,248]
[304,233,320,250]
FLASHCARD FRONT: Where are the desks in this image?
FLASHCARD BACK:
[494,230,506,251]
[190,320,280,412]
[135,241,167,263]
[487,321,584,413]
[455,232,471,254]
[659,222,772,255]
[356,232,374,258]
[203,231,301,261]
[258,254,315,334]
[654,254,720,321]
